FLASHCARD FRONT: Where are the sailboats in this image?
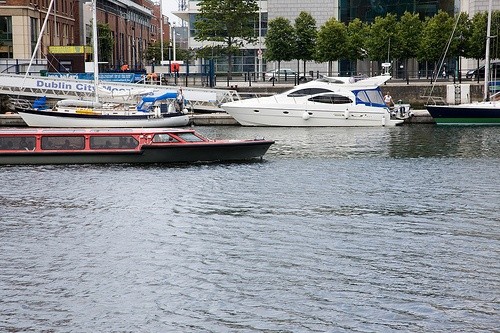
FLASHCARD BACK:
[11,2,197,130]
[425,0,500,129]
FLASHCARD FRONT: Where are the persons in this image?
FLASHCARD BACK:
[443,63,447,79]
[384,92,393,107]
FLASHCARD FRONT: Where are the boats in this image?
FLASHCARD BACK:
[0,125,276,164]
[223,77,415,128]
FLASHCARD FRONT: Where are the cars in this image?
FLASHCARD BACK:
[297,72,327,82]
[265,69,299,81]
[465,62,499,79]
